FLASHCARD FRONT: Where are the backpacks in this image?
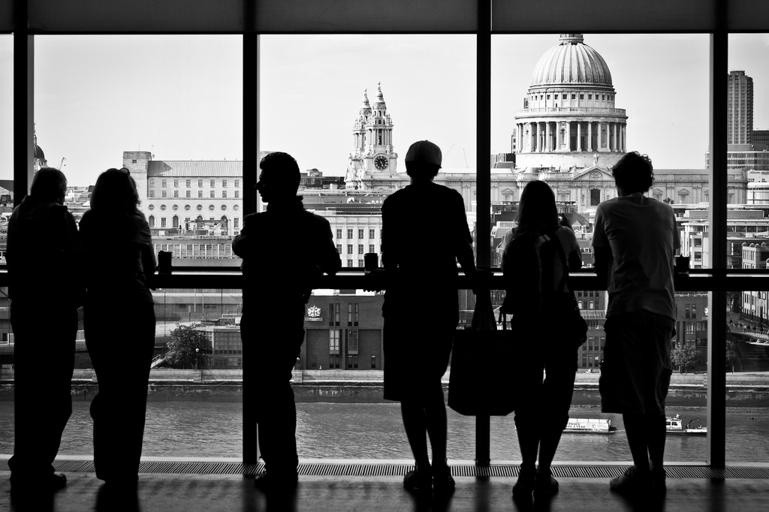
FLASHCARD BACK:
[497,229,580,332]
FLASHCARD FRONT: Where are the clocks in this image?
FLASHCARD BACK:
[372,152,390,173]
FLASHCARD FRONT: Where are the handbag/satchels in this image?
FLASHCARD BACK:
[448,288,513,417]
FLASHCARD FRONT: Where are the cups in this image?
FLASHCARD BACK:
[157,250,172,274]
[675,255,690,274]
[364,253,377,275]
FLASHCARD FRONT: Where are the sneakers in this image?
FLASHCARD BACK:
[404,465,669,506]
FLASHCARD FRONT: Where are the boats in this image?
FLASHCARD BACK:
[663,412,709,437]
[563,418,618,434]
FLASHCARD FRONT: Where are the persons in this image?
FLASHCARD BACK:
[499,180,588,499]
[590,152,683,507]
[381,140,480,502]
[232,152,341,500]
[80,169,172,486]
[6,168,79,492]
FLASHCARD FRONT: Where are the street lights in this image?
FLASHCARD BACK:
[194,347,200,371]
